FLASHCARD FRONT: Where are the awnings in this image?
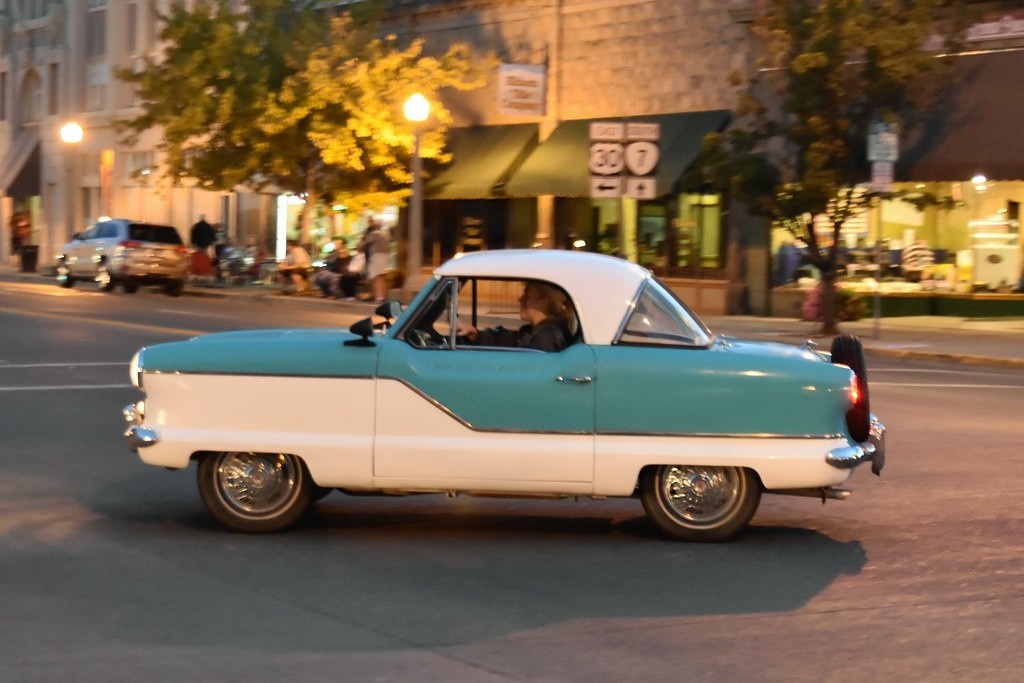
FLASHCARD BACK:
[0,127,43,200]
[421,109,731,198]
[684,39,1023,183]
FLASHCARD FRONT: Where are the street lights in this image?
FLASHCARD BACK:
[403,92,430,294]
[60,120,83,246]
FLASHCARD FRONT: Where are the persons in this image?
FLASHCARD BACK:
[317,215,392,305]
[190,214,229,263]
[277,239,315,295]
[455,281,577,353]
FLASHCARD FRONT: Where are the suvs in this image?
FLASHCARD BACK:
[53,219,191,296]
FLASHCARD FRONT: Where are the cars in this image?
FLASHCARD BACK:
[122,248,887,544]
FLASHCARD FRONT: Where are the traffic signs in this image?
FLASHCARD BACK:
[588,122,660,200]
